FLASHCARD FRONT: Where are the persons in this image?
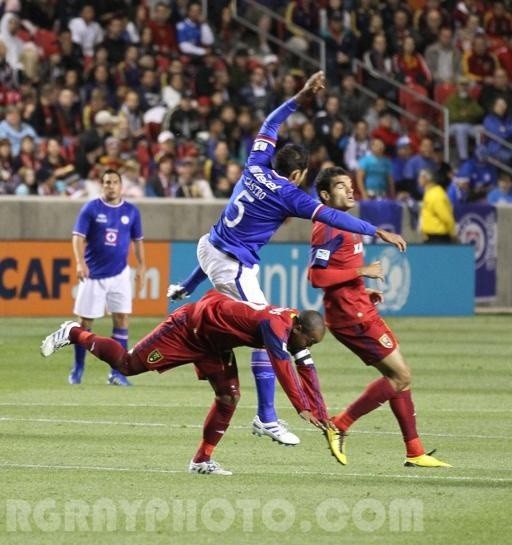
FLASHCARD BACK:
[305,163,452,469]
[416,166,458,244]
[167,70,408,447]
[67,168,147,391]
[38,291,326,478]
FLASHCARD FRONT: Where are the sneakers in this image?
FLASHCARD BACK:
[400,450,456,470]
[68,362,87,386]
[106,368,135,389]
[186,455,235,478]
[320,416,351,466]
[164,280,192,303]
[250,413,302,448]
[39,316,83,359]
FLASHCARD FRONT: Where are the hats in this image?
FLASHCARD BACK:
[155,128,177,144]
[92,109,124,127]
